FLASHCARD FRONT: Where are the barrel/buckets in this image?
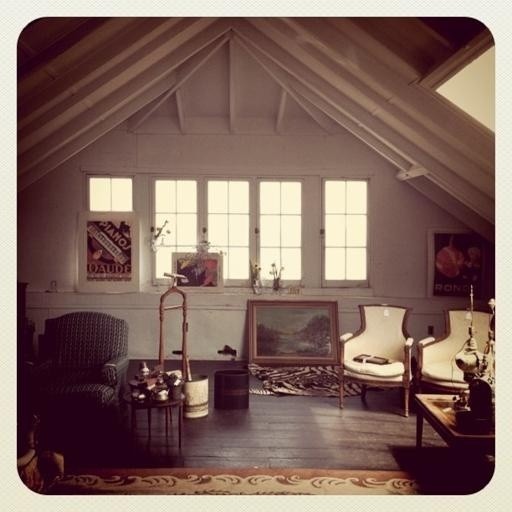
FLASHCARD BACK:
[183,374,208,420]
[213,369,249,410]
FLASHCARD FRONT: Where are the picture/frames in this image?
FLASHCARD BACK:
[171,252,223,290]
[75,212,140,297]
[428,227,494,302]
[246,299,341,366]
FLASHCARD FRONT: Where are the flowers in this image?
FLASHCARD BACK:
[249,260,261,283]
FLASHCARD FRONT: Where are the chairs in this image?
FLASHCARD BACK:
[338,303,494,419]
[24,311,128,411]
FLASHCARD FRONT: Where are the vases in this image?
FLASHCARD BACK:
[251,278,259,295]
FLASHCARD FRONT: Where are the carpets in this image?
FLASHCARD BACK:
[244,364,377,398]
[50,467,429,495]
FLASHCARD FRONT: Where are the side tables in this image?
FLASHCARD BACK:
[123,379,187,452]
